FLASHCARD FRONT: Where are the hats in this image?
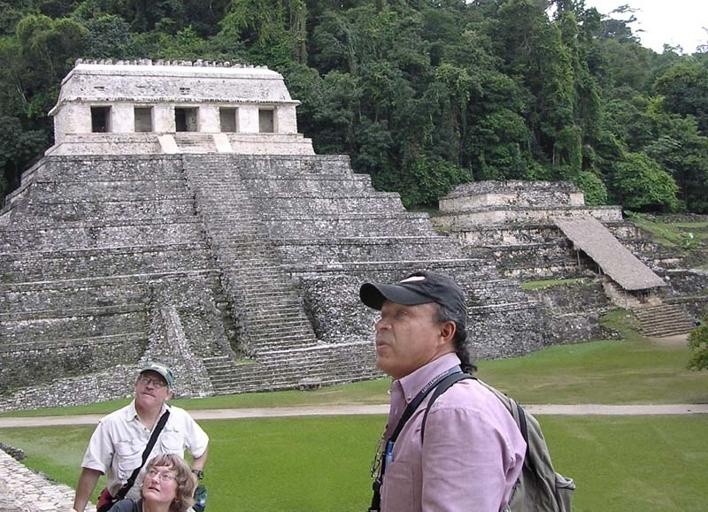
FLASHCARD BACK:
[357,268,469,317]
[137,362,175,388]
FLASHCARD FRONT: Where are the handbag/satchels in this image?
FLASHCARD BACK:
[192,484,210,512]
[92,486,115,512]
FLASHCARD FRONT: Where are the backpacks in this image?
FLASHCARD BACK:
[419,370,578,512]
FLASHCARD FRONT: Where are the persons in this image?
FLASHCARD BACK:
[359,270,526,512]
[70,364,209,512]
[105,453,195,512]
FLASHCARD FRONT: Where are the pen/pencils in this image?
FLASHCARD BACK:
[384,440,395,464]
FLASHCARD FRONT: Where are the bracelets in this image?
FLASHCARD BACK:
[191,470,203,480]
[73,507,78,512]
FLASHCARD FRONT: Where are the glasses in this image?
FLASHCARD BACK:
[137,374,169,391]
[146,467,177,483]
